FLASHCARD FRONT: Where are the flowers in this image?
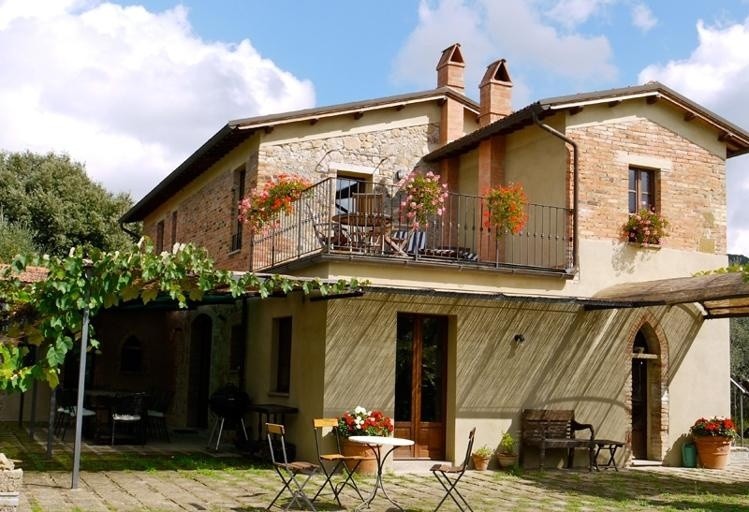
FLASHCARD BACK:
[236,175,314,232]
[624,209,670,245]
[393,166,450,229]
[692,416,739,441]
[335,406,392,437]
[477,181,527,234]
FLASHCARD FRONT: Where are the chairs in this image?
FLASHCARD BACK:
[430,426,478,512]
[203,382,251,453]
[52,378,184,448]
[309,416,371,508]
[263,422,320,510]
[304,187,418,259]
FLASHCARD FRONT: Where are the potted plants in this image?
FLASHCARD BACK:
[497,430,516,466]
[472,446,490,469]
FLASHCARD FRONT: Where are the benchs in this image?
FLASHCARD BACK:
[516,405,595,473]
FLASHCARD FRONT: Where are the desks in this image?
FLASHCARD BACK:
[250,403,300,462]
[592,438,626,471]
[347,433,417,512]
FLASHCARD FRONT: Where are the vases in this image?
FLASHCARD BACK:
[696,438,733,468]
[342,440,382,474]
